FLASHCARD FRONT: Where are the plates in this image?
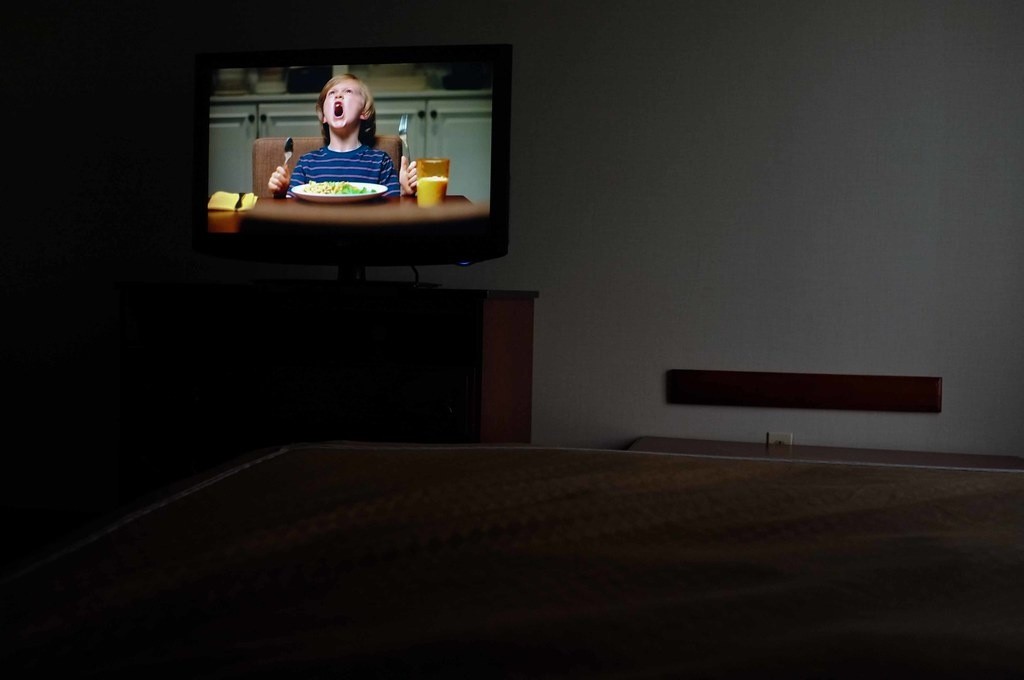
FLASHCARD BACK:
[291,182,389,204]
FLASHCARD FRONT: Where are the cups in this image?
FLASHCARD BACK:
[415,157,449,208]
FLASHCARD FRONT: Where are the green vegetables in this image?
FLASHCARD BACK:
[335,185,376,195]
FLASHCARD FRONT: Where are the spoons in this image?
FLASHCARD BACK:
[283,137,293,167]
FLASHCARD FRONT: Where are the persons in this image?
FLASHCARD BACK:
[268,74,418,199]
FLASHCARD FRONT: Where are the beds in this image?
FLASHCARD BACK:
[0,443,1023,679]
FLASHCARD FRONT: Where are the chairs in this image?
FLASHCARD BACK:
[253,136,403,199]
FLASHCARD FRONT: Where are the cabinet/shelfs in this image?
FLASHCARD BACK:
[210,98,493,202]
[113,281,538,505]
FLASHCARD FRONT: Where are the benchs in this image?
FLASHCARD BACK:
[628,437,1024,473]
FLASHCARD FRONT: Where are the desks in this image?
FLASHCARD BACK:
[254,195,476,212]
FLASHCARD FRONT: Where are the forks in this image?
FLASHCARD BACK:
[398,113,410,167]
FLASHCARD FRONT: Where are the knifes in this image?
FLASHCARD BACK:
[235,192,246,208]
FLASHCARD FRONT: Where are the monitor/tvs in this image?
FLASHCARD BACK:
[194,43,512,285]
